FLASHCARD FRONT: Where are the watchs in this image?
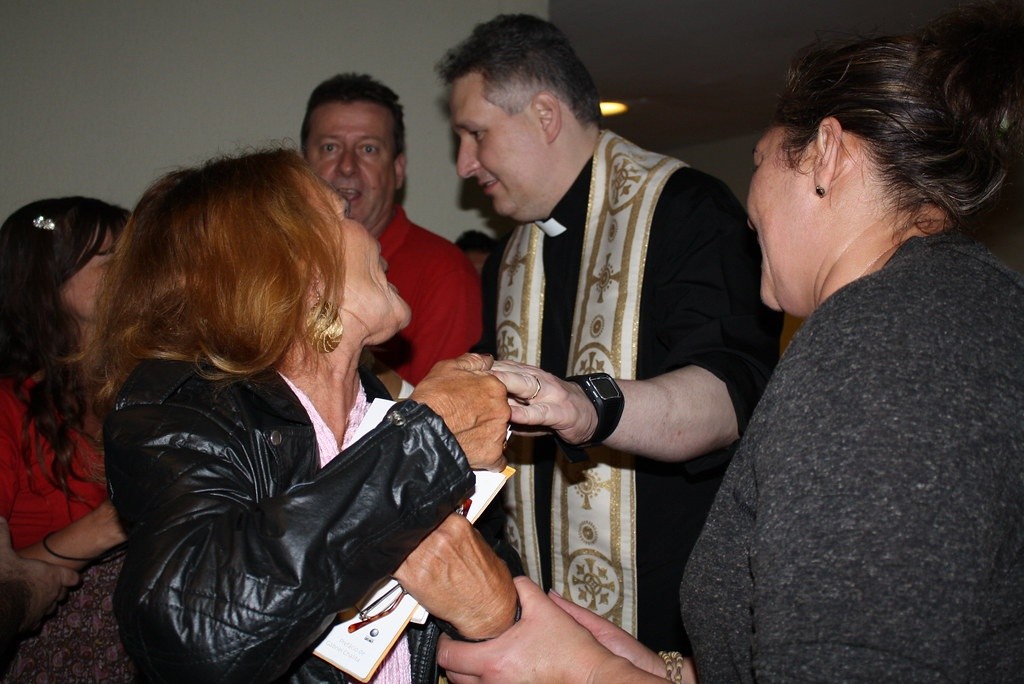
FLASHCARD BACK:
[550,373,625,463]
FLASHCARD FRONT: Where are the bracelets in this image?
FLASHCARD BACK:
[658,651,684,684]
[446,599,521,642]
[43,531,98,561]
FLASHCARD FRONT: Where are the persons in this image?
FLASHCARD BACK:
[437,0,1024,684]
[453,230,494,280]
[359,13,784,657]
[300,73,483,383]
[99,152,512,684]
[0,516,80,680]
[0,196,133,568]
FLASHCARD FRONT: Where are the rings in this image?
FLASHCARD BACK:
[515,376,541,402]
[505,425,511,443]
